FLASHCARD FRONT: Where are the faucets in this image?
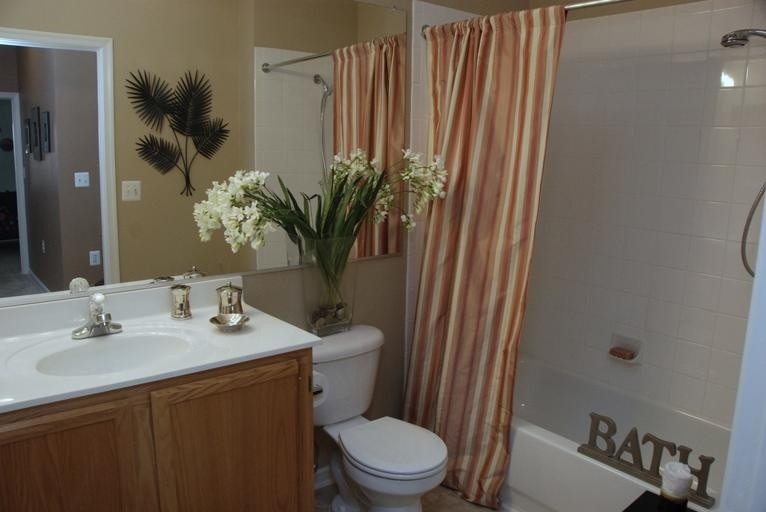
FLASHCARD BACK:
[72,292,123,340]
[69,278,90,292]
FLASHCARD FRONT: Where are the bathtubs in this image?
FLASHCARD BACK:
[499,355,731,512]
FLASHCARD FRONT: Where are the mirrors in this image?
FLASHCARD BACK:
[0,26,119,306]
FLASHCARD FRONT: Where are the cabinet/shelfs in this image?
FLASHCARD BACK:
[0,350,314,510]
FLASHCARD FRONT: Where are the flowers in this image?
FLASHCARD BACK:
[193,147,449,315]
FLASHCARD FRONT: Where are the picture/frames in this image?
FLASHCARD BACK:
[21,106,53,165]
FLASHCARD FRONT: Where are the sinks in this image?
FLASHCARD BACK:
[36,334,188,378]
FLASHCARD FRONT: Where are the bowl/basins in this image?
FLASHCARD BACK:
[210,314,250,332]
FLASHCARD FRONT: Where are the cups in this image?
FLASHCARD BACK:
[659,461,693,500]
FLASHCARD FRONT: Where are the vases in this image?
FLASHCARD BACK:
[298,234,359,338]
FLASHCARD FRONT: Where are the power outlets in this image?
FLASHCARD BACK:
[89,250,100,266]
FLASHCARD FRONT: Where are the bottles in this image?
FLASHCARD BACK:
[154,266,208,282]
[169,283,243,320]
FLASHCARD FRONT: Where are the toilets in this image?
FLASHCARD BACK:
[313,324,448,512]
